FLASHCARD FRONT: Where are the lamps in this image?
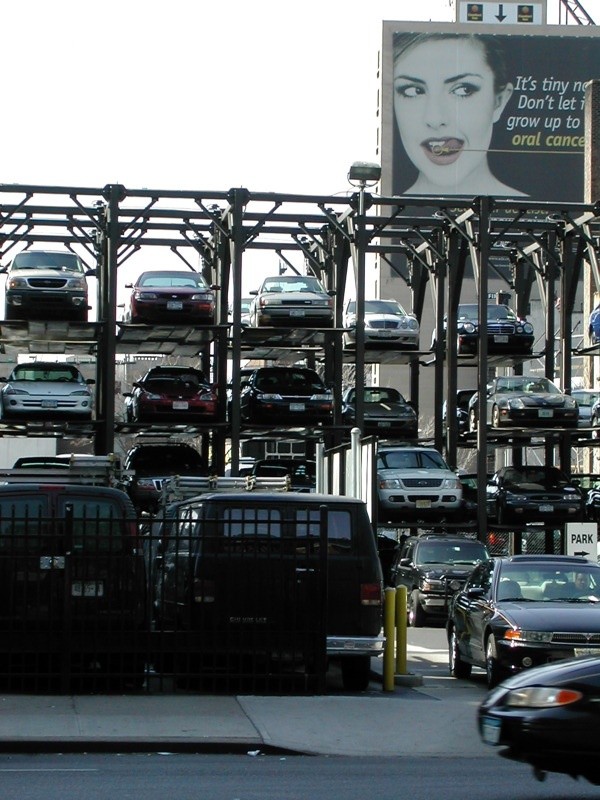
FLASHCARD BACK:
[347,161,382,187]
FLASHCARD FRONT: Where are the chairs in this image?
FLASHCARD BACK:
[497,580,522,600]
[557,582,577,598]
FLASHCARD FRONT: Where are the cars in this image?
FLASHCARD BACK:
[566,387,600,440]
[248,275,339,327]
[342,297,422,350]
[467,375,580,431]
[126,270,221,325]
[340,386,419,439]
[588,303,600,346]
[446,554,600,691]
[118,438,210,514]
[456,463,600,527]
[239,458,319,494]
[0,360,97,421]
[476,650,600,788]
[442,388,479,437]
[121,365,218,423]
[431,303,536,355]
[377,533,400,578]
[227,366,335,429]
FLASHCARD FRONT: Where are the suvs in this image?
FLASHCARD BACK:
[390,533,497,628]
[375,445,463,523]
[0,249,97,321]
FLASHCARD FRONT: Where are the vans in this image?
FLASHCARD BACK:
[139,490,386,688]
[0,484,147,694]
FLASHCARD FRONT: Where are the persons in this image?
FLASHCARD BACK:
[565,571,590,599]
[392,34,530,196]
